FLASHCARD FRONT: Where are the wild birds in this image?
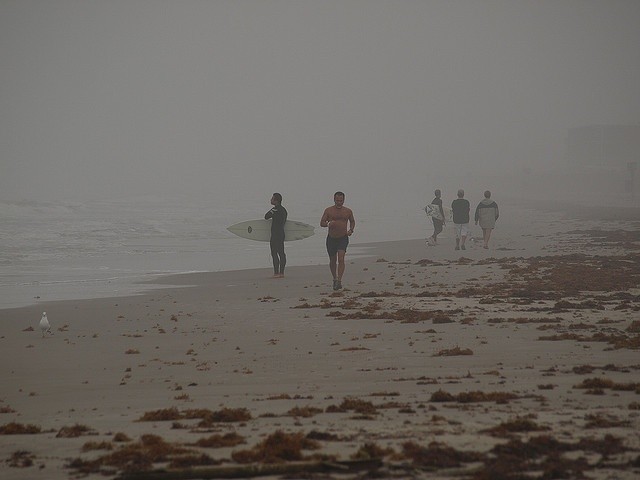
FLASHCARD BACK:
[37,311,52,338]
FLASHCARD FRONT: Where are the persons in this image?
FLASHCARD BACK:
[265,193,288,278]
[474,191,499,249]
[320,192,355,290]
[428,189,445,244]
[451,190,471,250]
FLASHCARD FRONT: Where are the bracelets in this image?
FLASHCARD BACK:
[327,222,330,227]
[350,229,354,233]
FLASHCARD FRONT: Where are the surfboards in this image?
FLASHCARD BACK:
[227,218,316,242]
[422,204,453,222]
